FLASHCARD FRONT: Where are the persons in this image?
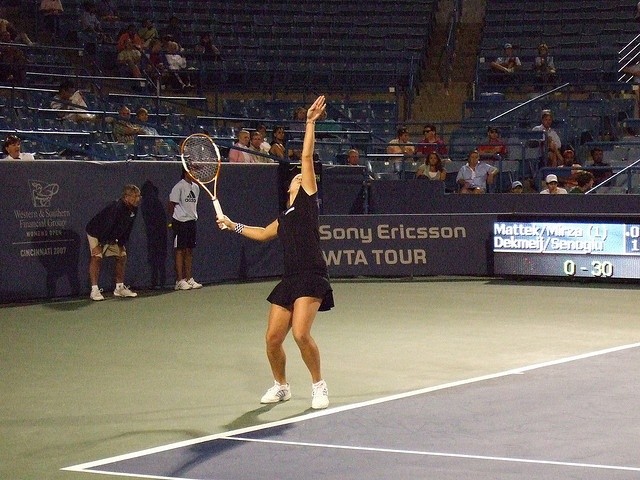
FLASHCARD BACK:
[228,130,256,163]
[0,135,35,161]
[62,28,80,65]
[255,125,290,161]
[160,17,183,44]
[386,127,415,162]
[132,107,179,155]
[0,31,13,51]
[113,105,146,145]
[248,131,275,163]
[347,149,360,166]
[464,107,474,119]
[475,125,509,159]
[416,152,447,182]
[520,177,538,194]
[511,180,524,194]
[490,43,522,73]
[316,108,328,121]
[141,39,174,92]
[117,38,142,78]
[0,7,39,52]
[534,43,555,68]
[563,163,584,191]
[269,127,286,149]
[215,95,334,409]
[163,40,192,90]
[583,146,613,194]
[293,106,306,121]
[137,18,159,42]
[556,149,582,168]
[540,173,567,194]
[194,33,220,55]
[67,78,93,123]
[85,184,138,301]
[600,116,619,141]
[580,130,595,144]
[116,24,143,52]
[50,79,77,110]
[455,151,498,193]
[36,13,58,46]
[38,0,65,14]
[9,68,28,87]
[413,124,448,164]
[569,170,596,194]
[169,162,203,290]
[80,4,101,33]
[532,109,562,167]
[78,42,98,67]
[616,111,627,131]
[98,0,118,16]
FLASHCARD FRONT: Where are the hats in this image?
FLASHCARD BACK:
[512,181,522,189]
[546,174,558,184]
[563,149,574,158]
[487,125,499,132]
[571,164,582,175]
[505,43,512,49]
[182,161,201,175]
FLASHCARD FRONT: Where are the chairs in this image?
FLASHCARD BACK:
[442,1,640,193]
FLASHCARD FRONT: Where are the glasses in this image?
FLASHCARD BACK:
[489,131,498,134]
[2,134,19,147]
[252,137,263,141]
[240,135,251,140]
[423,129,434,134]
[565,156,573,159]
[125,193,142,200]
[350,154,359,158]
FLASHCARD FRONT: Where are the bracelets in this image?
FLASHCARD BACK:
[235,223,244,235]
[98,243,103,248]
[305,122,316,126]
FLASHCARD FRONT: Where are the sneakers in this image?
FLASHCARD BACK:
[261,381,291,403]
[114,286,137,297]
[174,280,193,290]
[187,278,203,288]
[311,379,329,409]
[90,288,104,301]
